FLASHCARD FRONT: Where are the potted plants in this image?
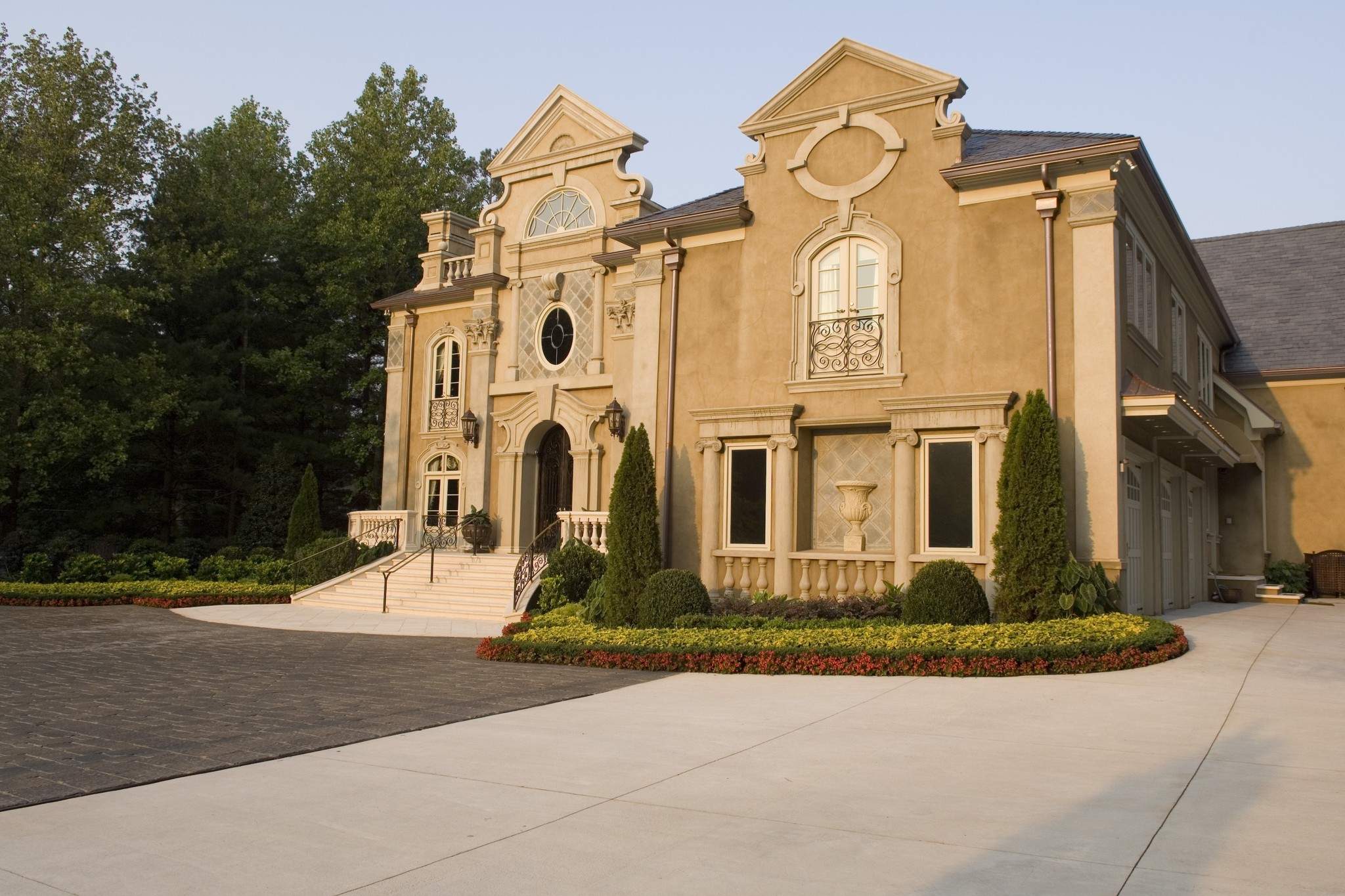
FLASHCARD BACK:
[575,507,605,544]
[463,504,492,553]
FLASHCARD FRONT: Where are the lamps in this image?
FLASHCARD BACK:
[606,397,626,443]
[1110,157,1139,172]
[461,406,482,448]
